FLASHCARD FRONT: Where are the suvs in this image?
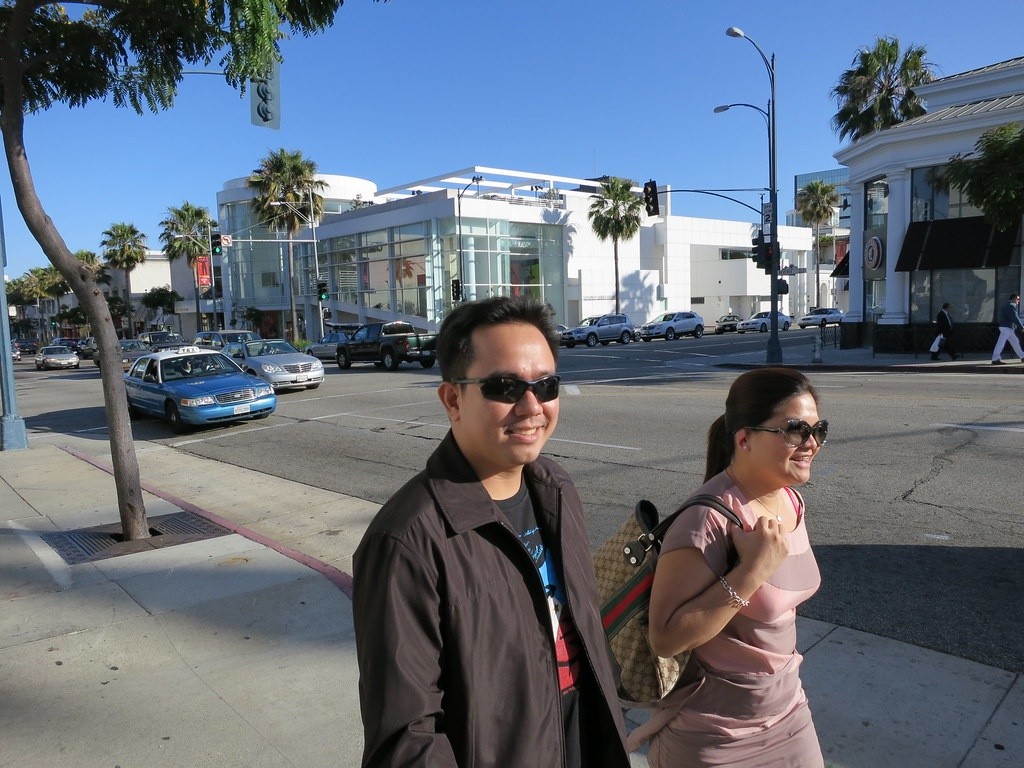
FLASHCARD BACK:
[553,313,641,348]
[640,312,704,342]
[136,331,192,353]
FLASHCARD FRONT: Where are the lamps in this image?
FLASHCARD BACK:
[412,190,423,196]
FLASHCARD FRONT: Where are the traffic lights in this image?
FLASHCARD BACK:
[317,283,328,301]
[643,181,659,216]
[452,279,460,300]
[777,279,788,294]
[752,231,764,268]
[211,233,222,256]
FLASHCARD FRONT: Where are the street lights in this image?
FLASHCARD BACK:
[270,187,325,339]
[713,27,782,363]
[457,175,485,301]
[175,215,217,331]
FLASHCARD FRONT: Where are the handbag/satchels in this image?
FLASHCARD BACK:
[930,333,943,352]
[599,493,744,753]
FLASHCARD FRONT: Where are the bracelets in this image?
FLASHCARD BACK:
[718,576,750,606]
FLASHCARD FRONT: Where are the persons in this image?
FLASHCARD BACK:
[991,293,1024,365]
[352,292,637,768]
[628,368,826,767]
[930,303,961,360]
[179,358,193,375]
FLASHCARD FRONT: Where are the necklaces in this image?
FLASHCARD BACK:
[732,467,782,521]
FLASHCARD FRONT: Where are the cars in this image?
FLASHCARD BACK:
[736,311,791,334]
[305,333,350,360]
[35,346,80,370]
[220,339,325,395]
[124,346,276,435]
[797,308,843,329]
[51,337,153,373]
[715,314,741,335]
[11,337,37,361]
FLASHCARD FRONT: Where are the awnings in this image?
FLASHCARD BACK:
[829,250,849,278]
[893,215,1018,272]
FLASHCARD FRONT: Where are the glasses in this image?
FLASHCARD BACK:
[455,376,561,403]
[1017,298,1020,300]
[744,420,829,448]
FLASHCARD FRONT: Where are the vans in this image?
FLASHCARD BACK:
[191,330,263,351]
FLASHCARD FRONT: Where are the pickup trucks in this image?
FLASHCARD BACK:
[336,322,438,371]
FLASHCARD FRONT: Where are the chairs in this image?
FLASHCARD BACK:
[164,363,183,380]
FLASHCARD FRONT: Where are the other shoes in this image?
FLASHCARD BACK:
[952,355,960,361]
[1022,357,1024,362]
[931,356,941,361]
[992,359,1005,365]
